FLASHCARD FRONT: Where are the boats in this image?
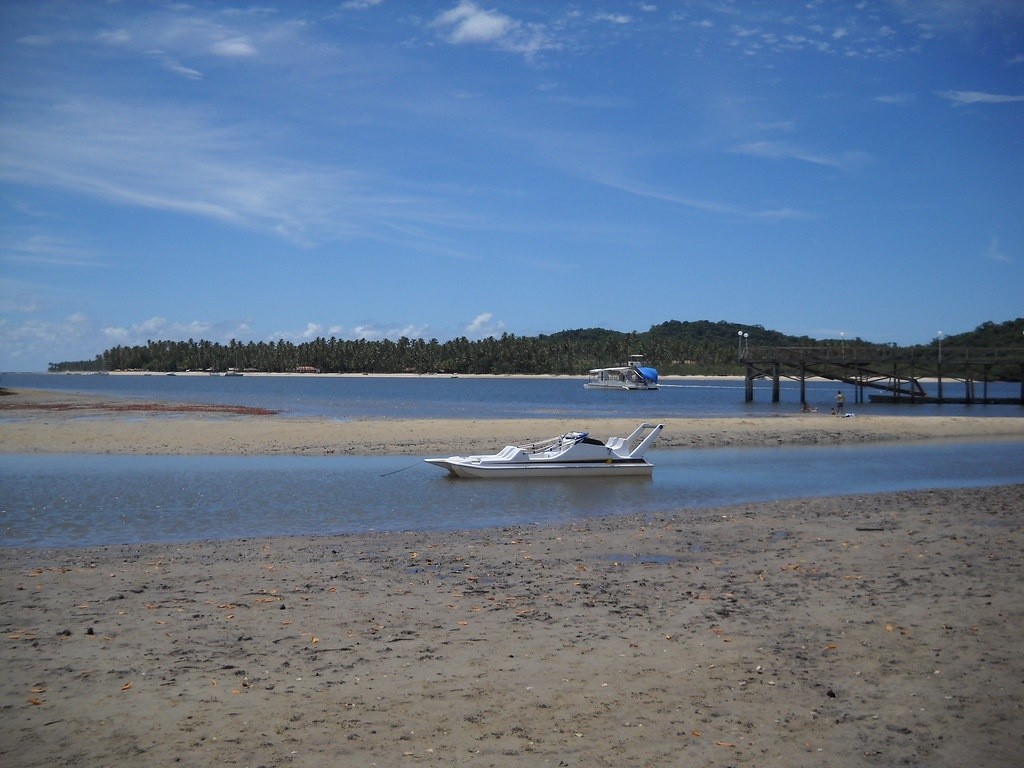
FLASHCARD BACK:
[167,373,176,376]
[583,367,659,391]
[424,423,666,478]
[144,373,151,376]
[210,373,220,376]
[451,376,458,378]
[225,373,243,376]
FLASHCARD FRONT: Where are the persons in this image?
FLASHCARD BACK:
[836,391,845,415]
[831,407,835,415]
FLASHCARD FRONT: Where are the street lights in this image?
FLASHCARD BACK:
[744,333,748,359]
[841,332,844,359]
[738,331,743,360]
[939,331,942,364]
